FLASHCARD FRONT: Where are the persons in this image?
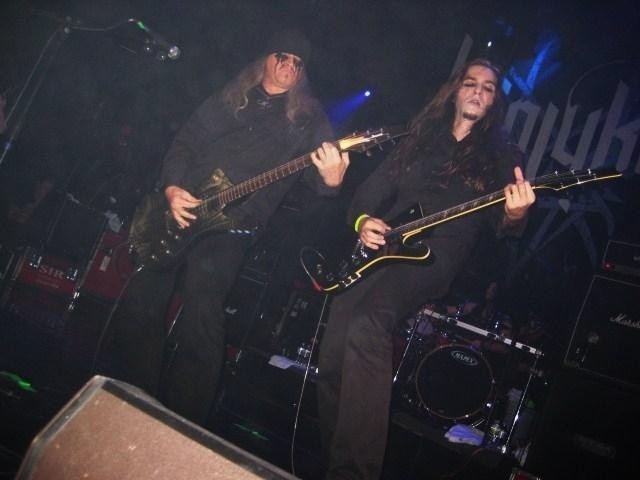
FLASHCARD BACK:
[120,29,350,427]
[313,59,536,479]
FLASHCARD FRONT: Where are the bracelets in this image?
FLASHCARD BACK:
[354,213,370,233]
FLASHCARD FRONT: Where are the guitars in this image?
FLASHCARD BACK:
[131,129,389,271]
[302,166,622,292]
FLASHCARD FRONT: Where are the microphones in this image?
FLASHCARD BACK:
[133,18,182,61]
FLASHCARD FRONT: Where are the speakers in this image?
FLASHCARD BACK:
[41,195,109,260]
[16,375,302,480]
[393,307,546,459]
[521,270,640,480]
[268,291,334,376]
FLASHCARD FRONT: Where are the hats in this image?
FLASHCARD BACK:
[251,27,311,66]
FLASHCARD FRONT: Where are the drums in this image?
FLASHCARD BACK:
[416,344,497,428]
